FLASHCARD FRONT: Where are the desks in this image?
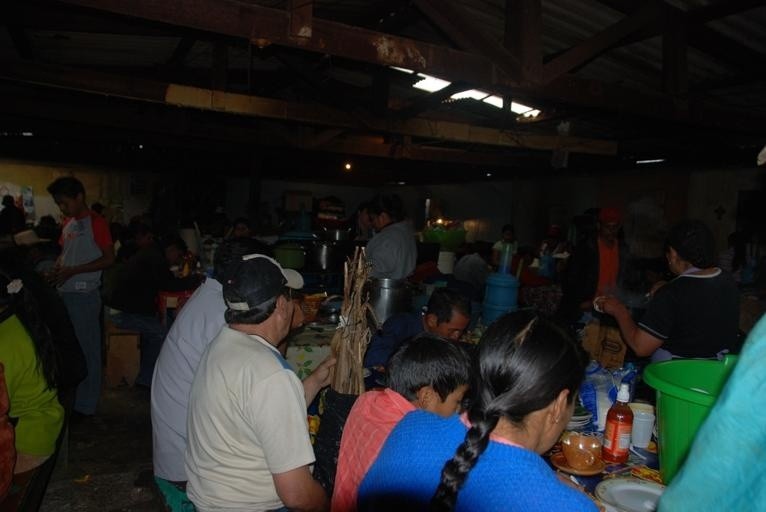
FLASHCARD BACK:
[284,290,345,447]
[154,250,215,342]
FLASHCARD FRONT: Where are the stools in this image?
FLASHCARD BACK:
[104,319,143,391]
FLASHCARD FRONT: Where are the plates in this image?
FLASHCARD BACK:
[550,454,606,476]
[594,478,669,511]
[566,412,594,428]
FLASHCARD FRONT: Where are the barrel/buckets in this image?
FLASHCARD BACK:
[485,266,516,323]
[642,353,741,486]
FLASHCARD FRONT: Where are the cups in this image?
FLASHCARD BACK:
[627,402,654,416]
[564,436,602,469]
[633,411,656,449]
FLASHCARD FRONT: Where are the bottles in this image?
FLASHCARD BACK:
[580,360,618,432]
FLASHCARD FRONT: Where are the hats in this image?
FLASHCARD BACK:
[599,208,622,220]
[222,253,305,312]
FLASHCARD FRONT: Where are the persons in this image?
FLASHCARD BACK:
[1,176,764,512]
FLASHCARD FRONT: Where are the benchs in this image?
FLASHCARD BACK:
[0,383,76,512]
